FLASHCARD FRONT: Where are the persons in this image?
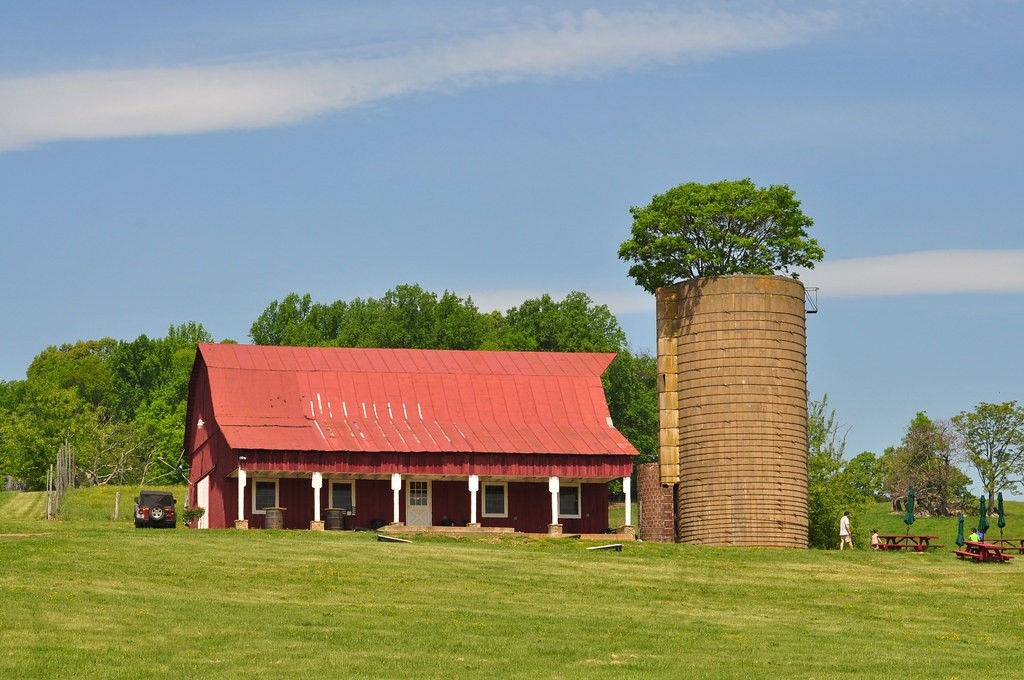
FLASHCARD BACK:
[839,511,855,550]
[870,529,882,551]
[968,529,980,562]
[970,526,983,540]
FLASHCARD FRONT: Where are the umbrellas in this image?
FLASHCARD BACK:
[956,513,964,552]
[904,488,916,549]
[998,491,1006,554]
[978,495,989,542]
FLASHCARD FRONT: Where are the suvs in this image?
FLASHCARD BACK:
[133,490,178,529]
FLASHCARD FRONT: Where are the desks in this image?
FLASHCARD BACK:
[878,534,939,552]
[963,541,1006,564]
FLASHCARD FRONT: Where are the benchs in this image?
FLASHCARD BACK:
[871,544,927,549]
[953,550,983,561]
[990,552,1015,561]
[928,545,944,549]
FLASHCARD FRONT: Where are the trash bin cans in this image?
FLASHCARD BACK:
[326,508,348,531]
[265,509,284,529]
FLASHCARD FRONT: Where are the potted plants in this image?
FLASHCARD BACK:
[181,506,205,529]
